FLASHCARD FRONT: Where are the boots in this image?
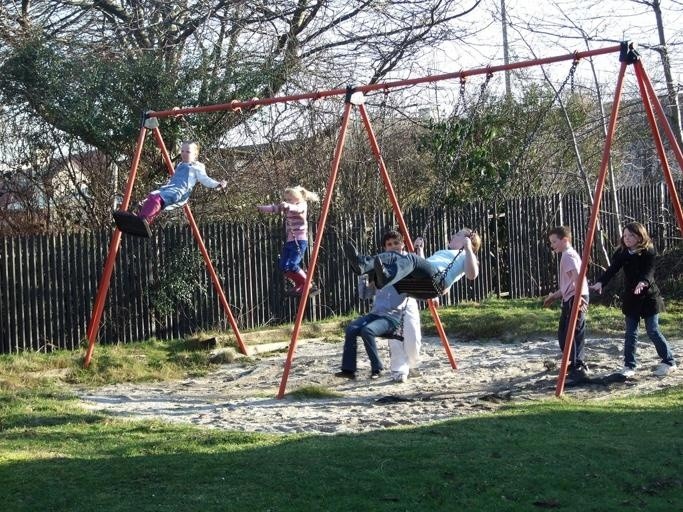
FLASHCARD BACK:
[293,268,313,293]
[285,272,296,295]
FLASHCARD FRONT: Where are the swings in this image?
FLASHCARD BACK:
[172,103,322,298]
[109,98,261,239]
[365,77,468,341]
[394,60,578,296]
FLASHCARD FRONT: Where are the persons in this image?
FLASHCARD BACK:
[590,222,676,380]
[137,141,228,230]
[541,227,590,385]
[334,250,408,381]
[343,227,480,299]
[254,186,320,295]
[371,231,422,383]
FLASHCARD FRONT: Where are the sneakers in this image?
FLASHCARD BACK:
[344,241,364,276]
[333,369,356,379]
[395,374,409,384]
[563,364,590,387]
[373,256,390,288]
[370,367,385,379]
[409,368,422,378]
[614,366,636,378]
[653,363,677,376]
